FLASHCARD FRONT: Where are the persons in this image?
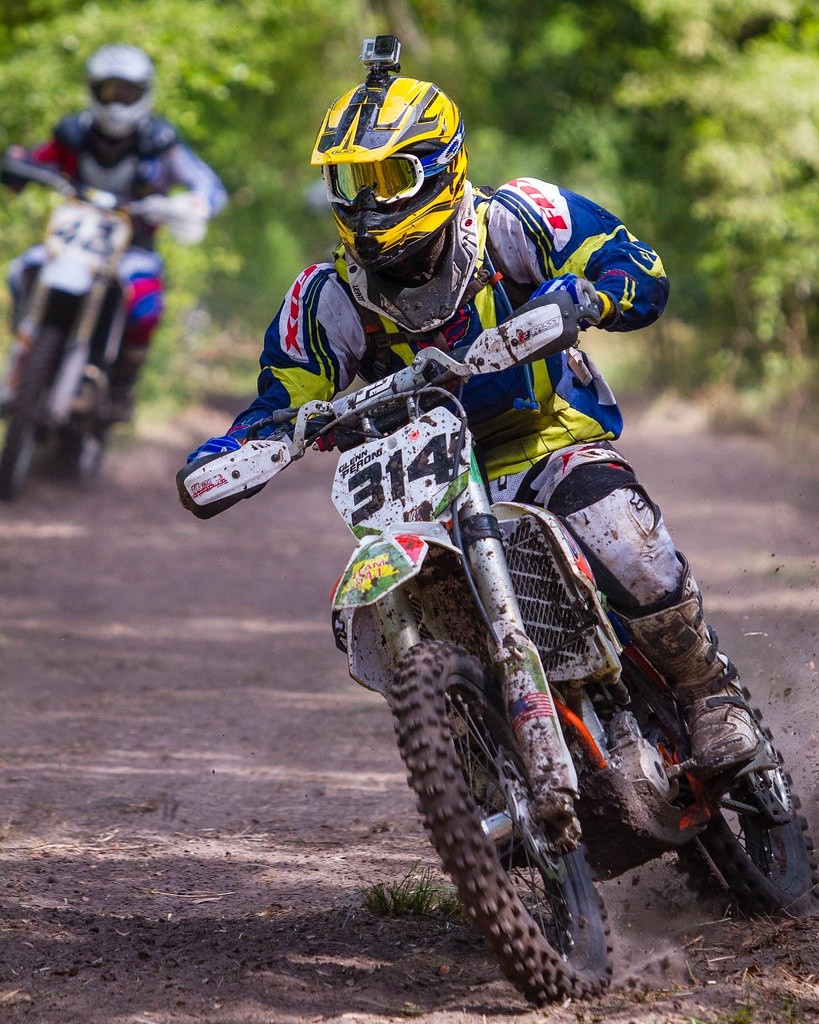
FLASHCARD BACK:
[175,76,766,872]
[0,43,226,422]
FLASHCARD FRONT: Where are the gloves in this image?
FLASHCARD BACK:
[528,273,598,331]
[186,435,243,464]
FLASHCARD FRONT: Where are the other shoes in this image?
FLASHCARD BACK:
[0,386,25,416]
[99,394,134,420]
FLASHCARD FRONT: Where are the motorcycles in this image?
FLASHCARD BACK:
[0,153,191,503]
[177,294,814,1003]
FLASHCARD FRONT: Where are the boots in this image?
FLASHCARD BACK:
[611,551,766,778]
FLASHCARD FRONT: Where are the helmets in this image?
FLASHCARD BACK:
[86,42,156,137]
[309,35,468,271]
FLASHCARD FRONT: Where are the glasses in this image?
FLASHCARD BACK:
[323,154,426,204]
[93,77,145,106]
